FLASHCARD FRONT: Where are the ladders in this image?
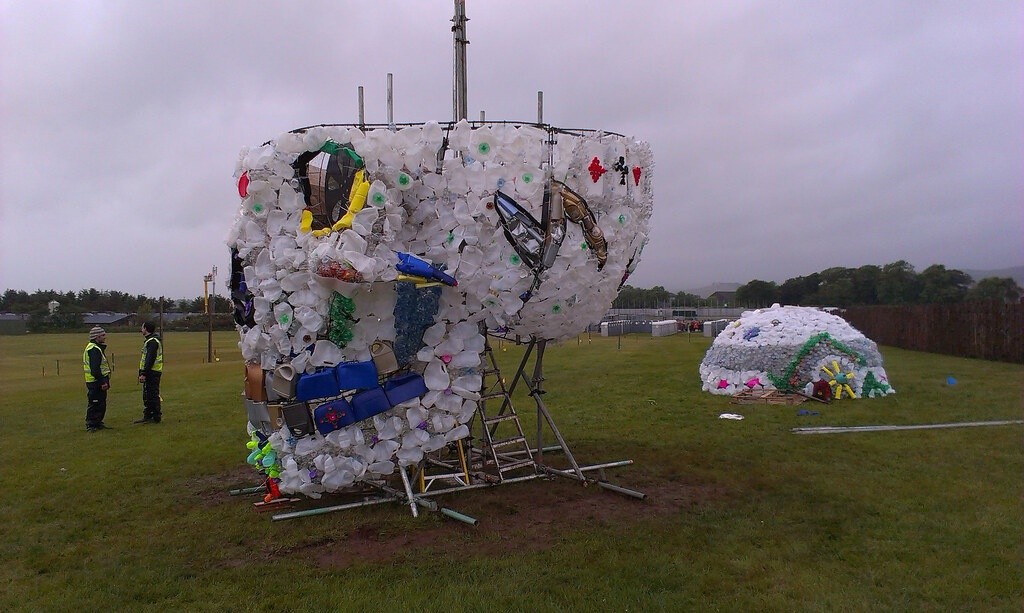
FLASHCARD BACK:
[446,319,538,484]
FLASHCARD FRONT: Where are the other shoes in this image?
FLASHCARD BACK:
[134,419,149,424]
[86,424,113,433]
[143,420,161,424]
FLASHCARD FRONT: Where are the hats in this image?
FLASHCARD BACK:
[90,325,105,340]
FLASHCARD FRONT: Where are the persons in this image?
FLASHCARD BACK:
[82,326,113,432]
[679,319,715,333]
[132,320,164,425]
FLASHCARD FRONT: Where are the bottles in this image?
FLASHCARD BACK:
[227,117,655,499]
[699,301,896,401]
